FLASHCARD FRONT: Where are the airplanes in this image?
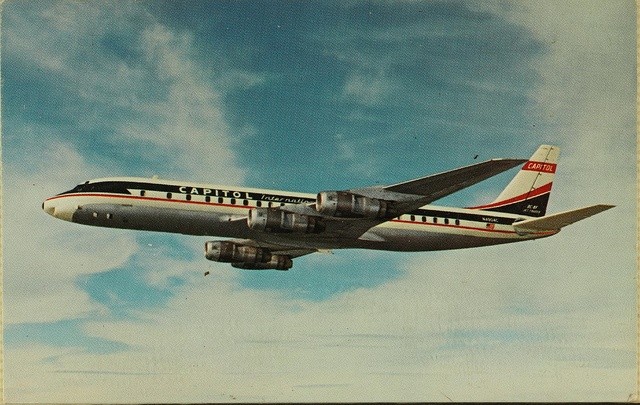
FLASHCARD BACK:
[40,143,616,271]
